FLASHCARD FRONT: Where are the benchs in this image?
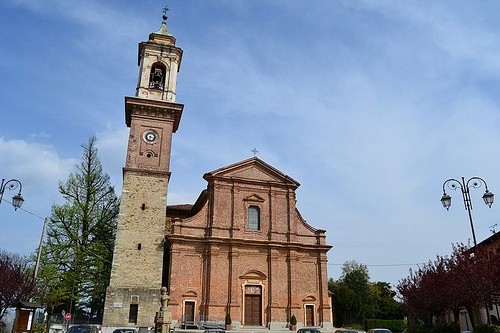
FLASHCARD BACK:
[185,321,225,331]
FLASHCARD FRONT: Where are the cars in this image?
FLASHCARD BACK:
[49,322,392,333]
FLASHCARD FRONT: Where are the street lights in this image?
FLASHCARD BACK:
[0,178,25,211]
[440,176,495,323]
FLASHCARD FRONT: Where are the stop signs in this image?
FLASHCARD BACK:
[65,313,71,320]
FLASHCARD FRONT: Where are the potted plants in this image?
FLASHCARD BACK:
[289,315,297,332]
[226,313,232,330]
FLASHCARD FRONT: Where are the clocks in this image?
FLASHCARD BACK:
[147,133,156,142]
